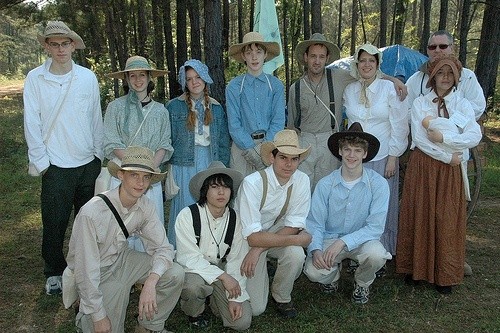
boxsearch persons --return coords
[236,130,312,316]
[61,147,185,333]
[341,42,409,275]
[285,32,408,193]
[421,115,460,133]
[23,21,103,295]
[102,55,173,254]
[410,52,483,287]
[404,30,487,275]
[174,160,253,330]
[225,33,286,184]
[163,58,232,251]
[303,122,391,305]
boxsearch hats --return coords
[295,32,341,68]
[327,121,380,163]
[107,146,167,184]
[36,21,86,49]
[105,56,172,79]
[349,43,383,109]
[228,32,281,64]
[188,161,243,202]
[261,129,311,168]
[178,59,213,92]
[426,54,463,118]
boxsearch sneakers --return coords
[46,275,62,295]
[319,281,338,293]
[352,278,370,304]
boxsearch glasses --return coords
[428,42,453,51]
[45,41,73,48]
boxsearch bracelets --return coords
[441,134,444,143]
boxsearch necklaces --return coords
[205,204,228,259]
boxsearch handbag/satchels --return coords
[28,159,40,176]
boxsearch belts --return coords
[251,134,264,139]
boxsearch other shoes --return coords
[269,290,298,318]
[462,262,473,275]
[346,257,359,272]
[133,324,175,333]
[188,316,210,328]
[417,279,430,288]
[437,285,452,293]
[377,264,387,278]
